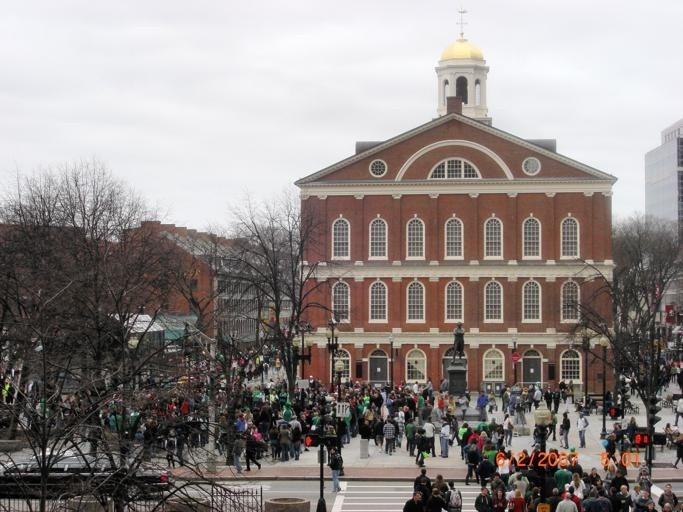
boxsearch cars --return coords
[1,447,170,500]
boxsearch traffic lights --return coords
[632,432,647,445]
[649,395,662,426]
[304,434,319,447]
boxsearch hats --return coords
[403,406,409,412]
[470,438,478,443]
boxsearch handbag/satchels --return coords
[339,466,344,476]
[528,500,535,509]
[508,422,513,430]
[507,501,514,511]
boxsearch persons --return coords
[0,334,681,512]
[451,321,465,364]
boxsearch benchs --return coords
[577,393,640,416]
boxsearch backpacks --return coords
[447,489,462,509]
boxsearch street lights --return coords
[292,322,315,414]
[389,333,395,393]
[599,330,609,440]
[511,333,519,388]
[335,356,345,462]
[325,318,342,394]
[582,326,591,417]
